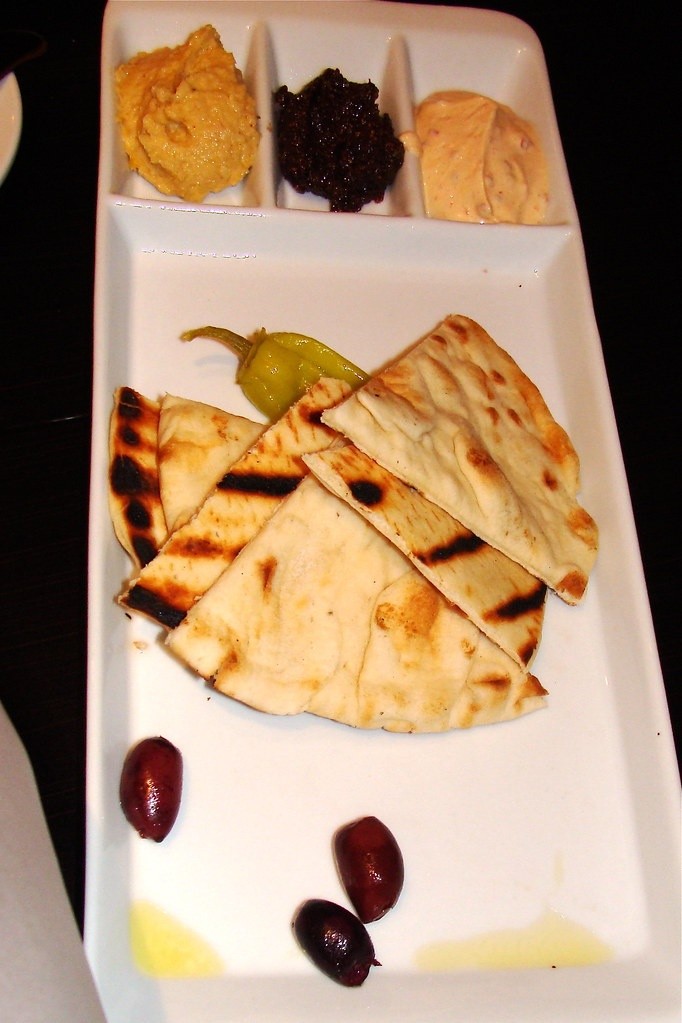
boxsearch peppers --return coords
[180,324,371,427]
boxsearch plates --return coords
[80,0,682,1023]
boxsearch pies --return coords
[105,312,599,732]
